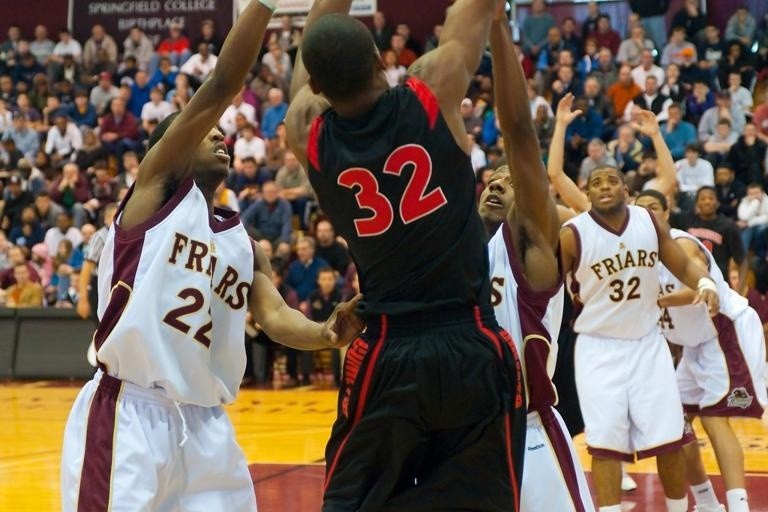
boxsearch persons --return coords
[558,163,719,512]
[633,185,768,512]
[278,0,529,512]
[475,0,595,512]
[548,280,588,442]
[539,86,683,496]
[56,0,367,512]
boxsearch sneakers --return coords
[241,375,336,386]
[598,471,637,511]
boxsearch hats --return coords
[680,47,694,55]
[31,243,48,258]
[461,98,471,106]
[98,70,113,78]
[168,24,182,30]
[7,175,20,185]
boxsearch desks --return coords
[2,305,100,381]
